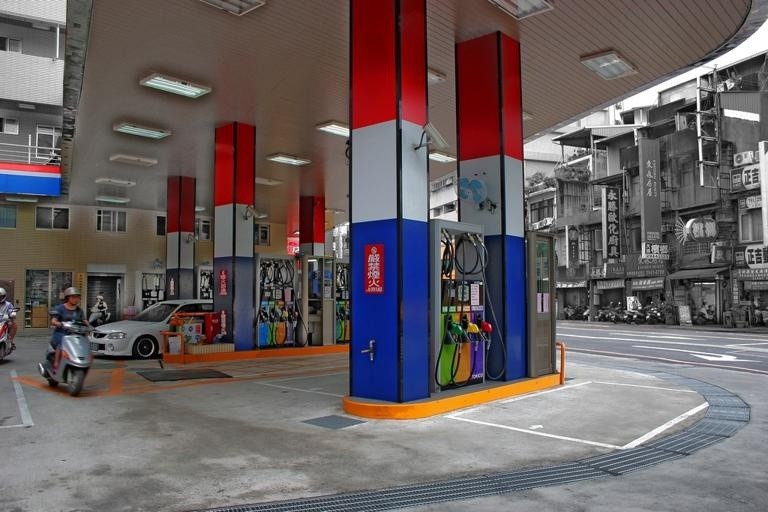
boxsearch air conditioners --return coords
[732,151,754,167]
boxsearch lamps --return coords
[94,72,213,205]
[255,119,348,186]
[491,1,555,21]
[428,150,456,164]
[425,68,448,89]
[521,112,536,122]
[580,50,640,81]
[199,0,265,18]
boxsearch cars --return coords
[89,297,215,358]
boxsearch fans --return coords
[452,176,497,214]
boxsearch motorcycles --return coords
[86,308,112,326]
[693,301,717,326]
[38,318,96,395]
[0,297,20,362]
[564,297,667,325]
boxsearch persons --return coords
[1,288,16,348]
[49,285,87,366]
[92,295,108,322]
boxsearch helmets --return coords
[63,287,81,298]
[0,287,7,302]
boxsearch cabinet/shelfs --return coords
[25,302,31,328]
[31,306,48,328]
